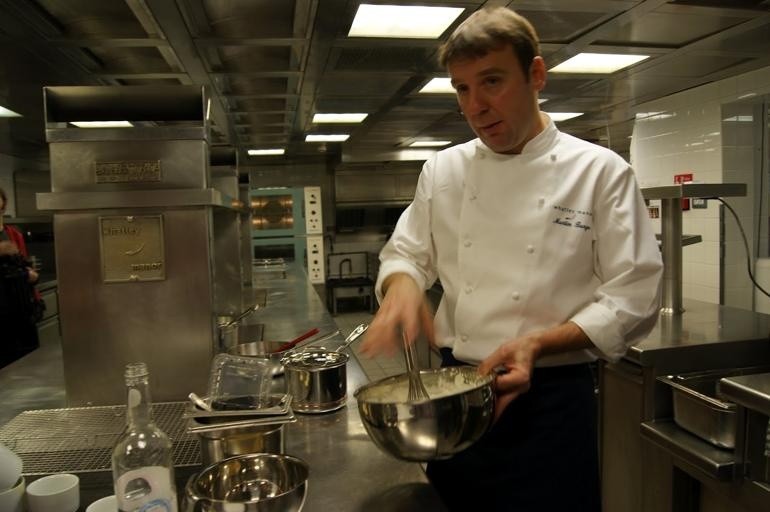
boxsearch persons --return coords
[356,6,667,511]
[0,186,42,302]
[0,215,42,369]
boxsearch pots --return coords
[228,331,338,376]
[218,304,265,350]
[282,323,369,413]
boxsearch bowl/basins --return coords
[353,366,496,462]
[181,452,310,512]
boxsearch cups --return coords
[26,473,80,512]
[0,477,27,512]
[0,444,22,490]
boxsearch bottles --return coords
[112,363,178,512]
